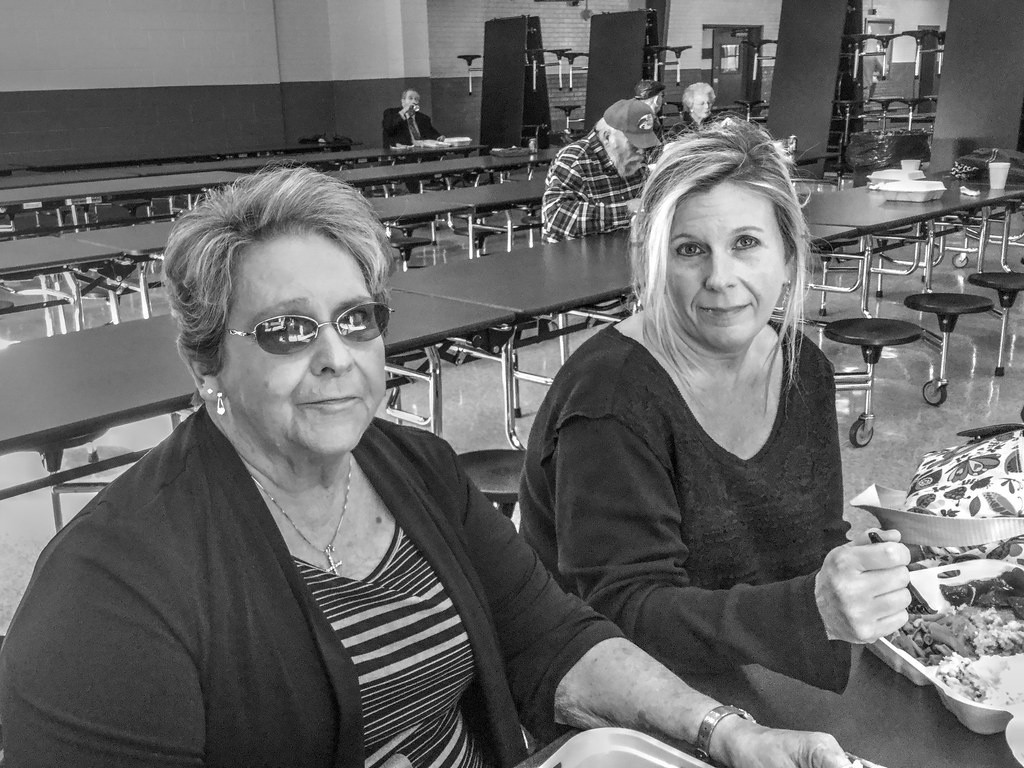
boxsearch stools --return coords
[1,28,1024,520]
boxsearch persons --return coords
[541,98,663,249]
[633,79,666,163]
[382,88,446,194]
[518,117,914,693]
[667,82,720,139]
[0,165,860,768]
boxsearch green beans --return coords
[888,608,974,664]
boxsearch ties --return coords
[407,117,420,140]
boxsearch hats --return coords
[633,80,666,99]
[606,98,661,149]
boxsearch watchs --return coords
[695,704,757,763]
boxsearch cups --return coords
[989,162,1011,190]
[899,159,922,171]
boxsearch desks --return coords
[765,0,849,179]
[0,133,362,175]
[0,146,565,241]
[927,0,1024,175]
[583,9,647,137]
[0,175,547,338]
[0,139,475,190]
[1,170,1024,520]
[478,14,528,157]
[512,640,1022,768]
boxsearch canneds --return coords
[529,138,538,153]
[413,104,420,112]
[789,135,797,152]
[631,210,645,244]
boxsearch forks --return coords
[869,531,938,621]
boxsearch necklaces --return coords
[250,451,353,576]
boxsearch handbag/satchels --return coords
[849,422,1024,566]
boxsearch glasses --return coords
[225,299,395,356]
[660,92,666,98]
